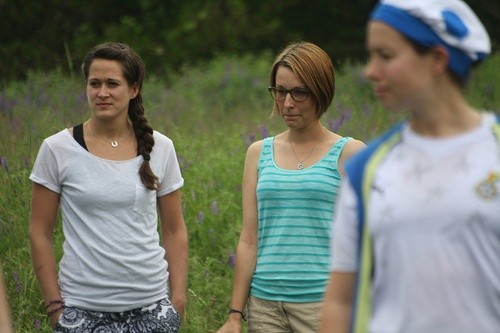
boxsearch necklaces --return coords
[287,126,326,170]
[86,118,132,147]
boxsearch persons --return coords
[0,264,12,333]
[216,41,368,333]
[29,42,189,333]
[320,0,500,333]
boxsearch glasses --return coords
[268,87,313,102]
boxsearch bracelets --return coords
[229,310,247,321]
[48,306,65,316]
[45,300,64,309]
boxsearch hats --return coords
[370,1,490,85]
